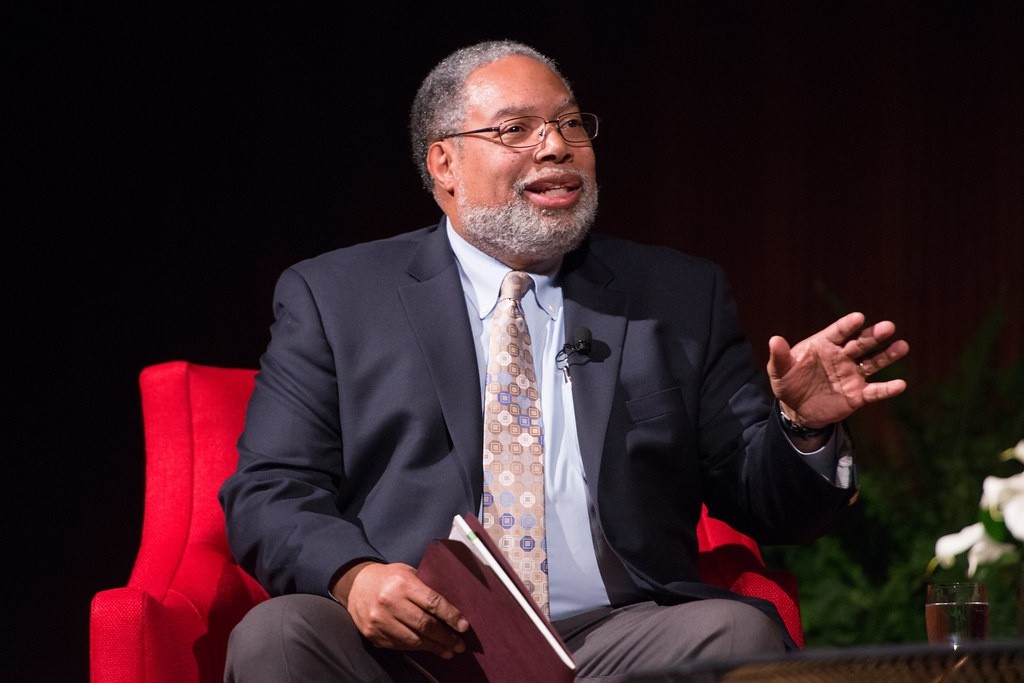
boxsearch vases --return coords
[925,582,988,646]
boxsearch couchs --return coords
[88,362,261,682]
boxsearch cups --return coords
[925,582,991,647]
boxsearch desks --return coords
[619,641,1024,683]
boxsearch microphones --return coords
[573,327,592,354]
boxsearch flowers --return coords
[930,443,1024,600]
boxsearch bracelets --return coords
[776,401,830,441]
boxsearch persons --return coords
[218,39,910,683]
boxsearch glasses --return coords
[441,112,602,148]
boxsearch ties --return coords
[483,272,552,629]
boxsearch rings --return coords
[859,359,871,376]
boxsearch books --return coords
[397,511,576,683]
